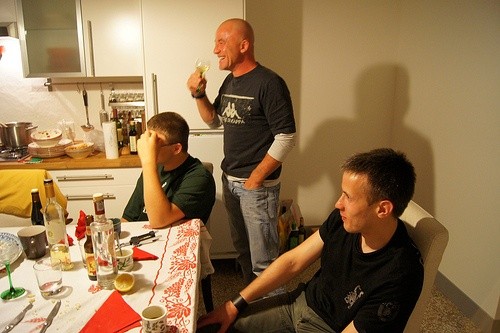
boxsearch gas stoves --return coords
[0,142,30,162]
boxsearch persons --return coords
[195,148,424,333]
[120,113,216,229]
[188,18,296,301]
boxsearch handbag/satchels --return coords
[277,199,297,253]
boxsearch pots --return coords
[0,121,38,147]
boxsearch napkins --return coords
[132,247,158,261]
[79,290,142,333]
[75,209,88,239]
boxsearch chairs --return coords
[399,200,449,333]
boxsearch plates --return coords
[26,139,72,158]
[0,231,23,273]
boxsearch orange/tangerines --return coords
[114,273,135,293]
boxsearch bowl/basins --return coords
[65,143,94,160]
[31,130,63,146]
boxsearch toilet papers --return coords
[102,120,119,160]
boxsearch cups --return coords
[141,305,167,333]
[115,249,134,270]
[18,225,46,258]
[107,218,121,239]
[79,238,88,268]
[33,257,63,296]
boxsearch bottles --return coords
[90,193,119,290]
[30,189,45,225]
[42,179,72,268]
[83,215,93,281]
[112,107,146,155]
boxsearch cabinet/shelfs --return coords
[47,0,247,275]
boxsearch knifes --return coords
[40,299,60,333]
[2,303,32,333]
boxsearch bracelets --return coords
[230,292,248,313]
[192,92,206,99]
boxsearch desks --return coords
[0,218,213,333]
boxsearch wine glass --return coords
[0,240,26,300]
[190,58,210,94]
